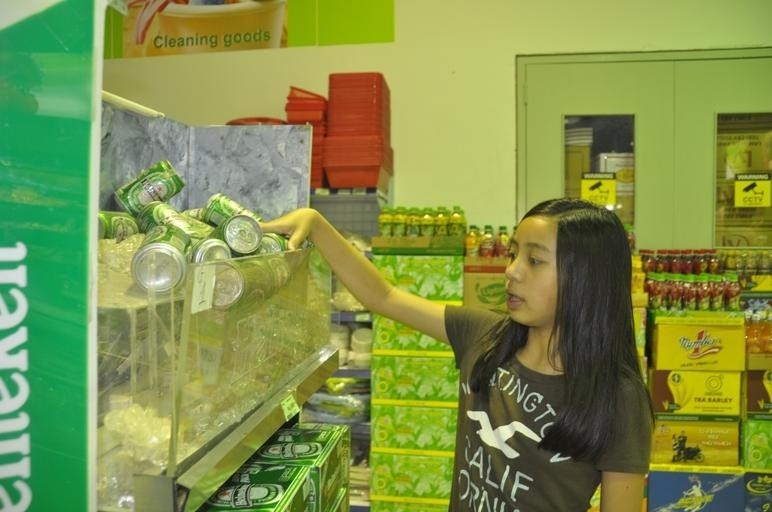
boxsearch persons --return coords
[254,194,654,512]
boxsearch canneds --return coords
[98,159,299,308]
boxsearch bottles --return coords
[639,248,771,310]
[377,206,517,258]
[742,298,772,354]
[624,224,635,256]
